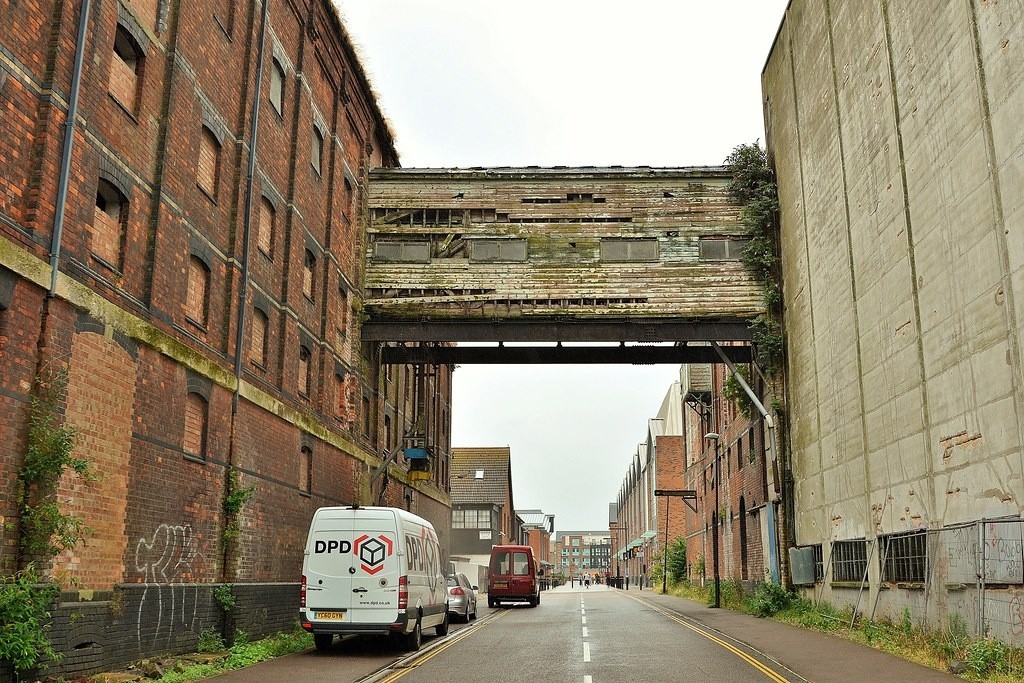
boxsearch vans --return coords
[488,544,544,608]
[300,506,455,652]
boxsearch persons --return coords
[584,572,589,589]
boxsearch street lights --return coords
[704,432,720,609]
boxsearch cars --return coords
[446,571,478,624]
